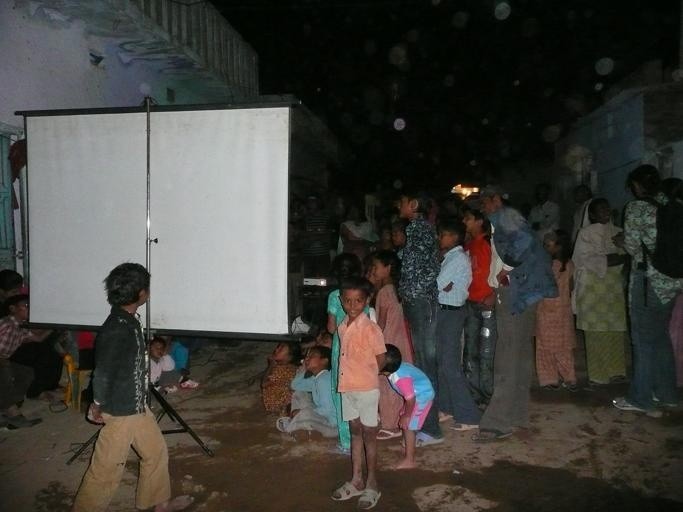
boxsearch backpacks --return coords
[636,193,683,280]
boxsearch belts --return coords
[439,304,461,312]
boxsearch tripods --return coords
[65,99,215,464]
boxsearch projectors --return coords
[303,275,333,286]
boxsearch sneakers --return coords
[179,380,200,390]
[611,396,664,420]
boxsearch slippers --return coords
[357,488,383,511]
[471,427,512,442]
[401,432,447,450]
[376,427,403,440]
[329,480,365,502]
[439,412,480,432]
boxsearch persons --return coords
[149,337,174,385]
[0,270,22,302]
[331,276,386,511]
[71,263,194,511]
[156,335,199,394]
[48,328,71,377]
[0,294,54,430]
